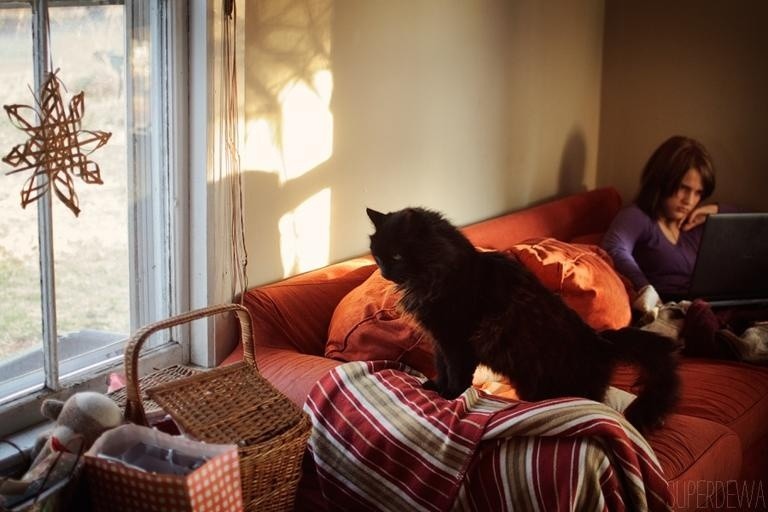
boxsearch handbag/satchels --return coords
[86,425,244,512]
[0,434,86,512]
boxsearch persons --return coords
[601,136,756,312]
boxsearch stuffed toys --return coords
[0,392,124,512]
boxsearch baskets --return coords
[102,303,314,512]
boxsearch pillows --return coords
[505,230,638,334]
[316,242,499,393]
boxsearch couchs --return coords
[217,184,767,512]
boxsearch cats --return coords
[364,204,683,434]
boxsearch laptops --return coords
[675,212,768,308]
